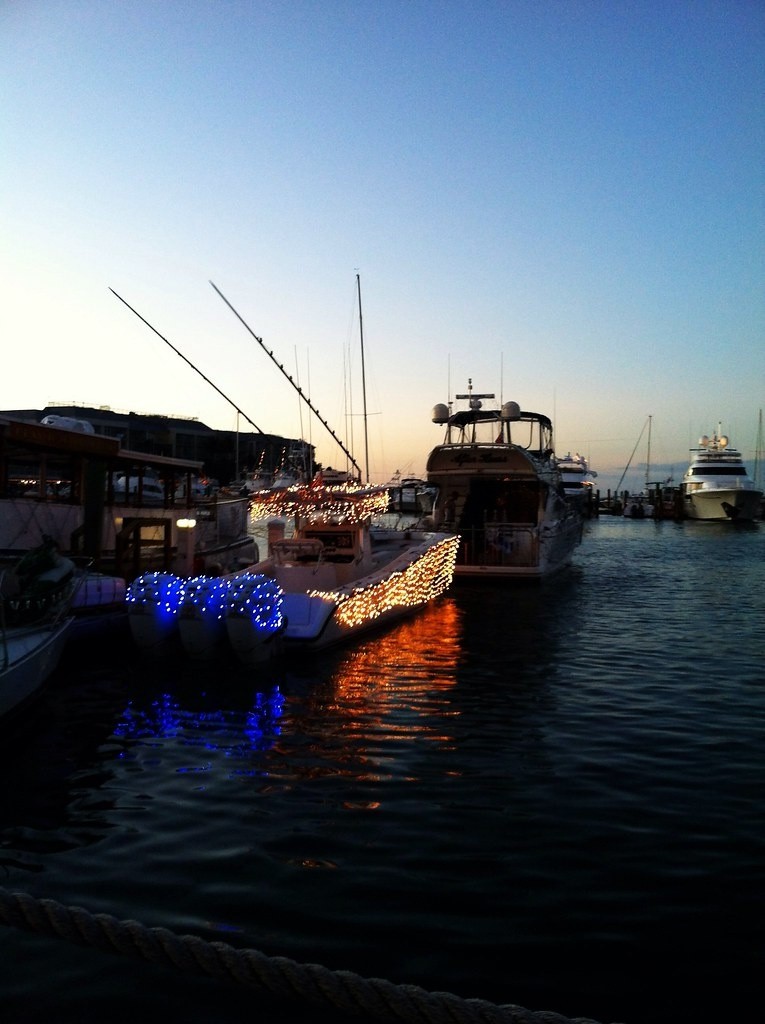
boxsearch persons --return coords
[439,491,457,537]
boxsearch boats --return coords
[0,280,765,726]
